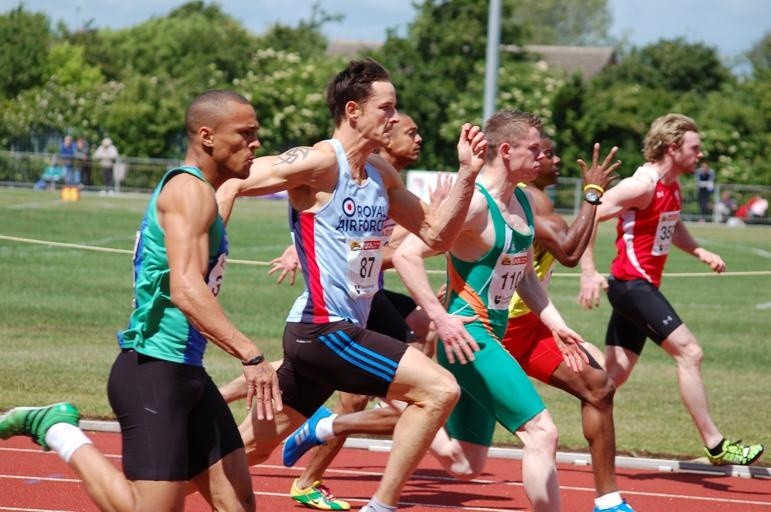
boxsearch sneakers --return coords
[282,404,333,466]
[704,439,764,466]
[0,401,81,451]
[594,497,634,512]
[290,478,350,511]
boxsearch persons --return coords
[695,161,768,223]
[0,87,282,511]
[578,113,765,464]
[33,132,118,194]
[215,57,634,511]
